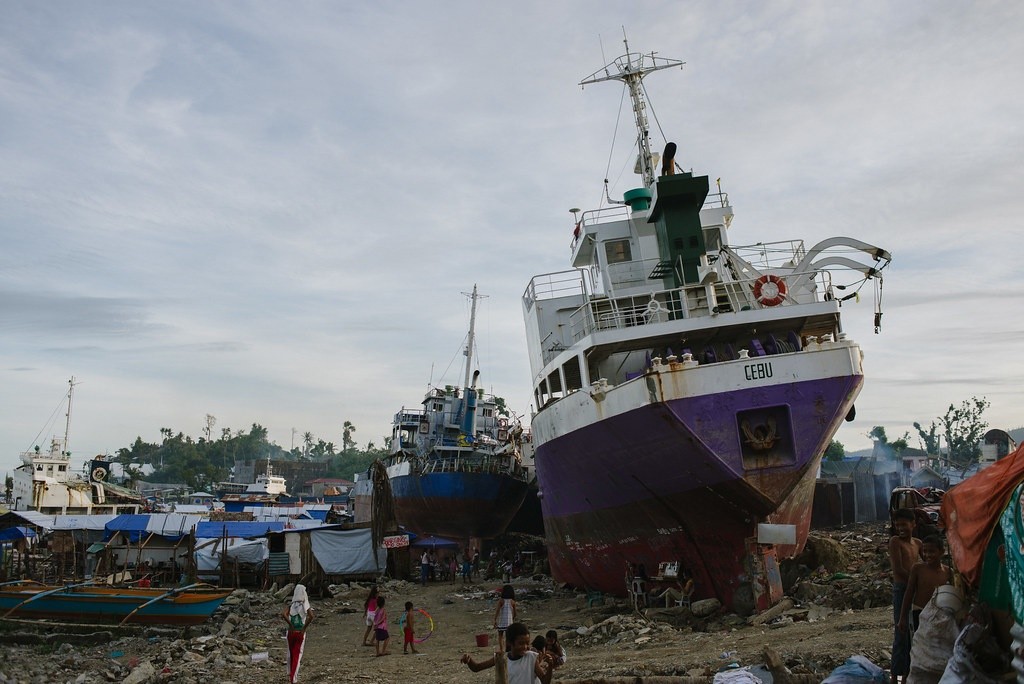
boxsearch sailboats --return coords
[245,453,287,496]
[11,375,140,517]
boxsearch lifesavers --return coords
[752,275,786,306]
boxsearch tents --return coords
[413,538,460,563]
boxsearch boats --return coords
[0,577,236,627]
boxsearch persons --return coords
[530,630,566,671]
[421,545,516,586]
[404,601,419,654]
[169,558,178,581]
[494,585,517,654]
[896,535,954,637]
[373,597,390,656]
[121,507,132,514]
[96,509,101,514]
[255,559,269,589]
[283,585,314,684]
[461,622,553,684]
[145,561,152,571]
[889,508,926,684]
[138,498,158,514]
[362,585,378,646]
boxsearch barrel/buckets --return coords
[476,634,488,647]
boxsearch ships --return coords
[522,23,895,616]
[370,282,541,584]
